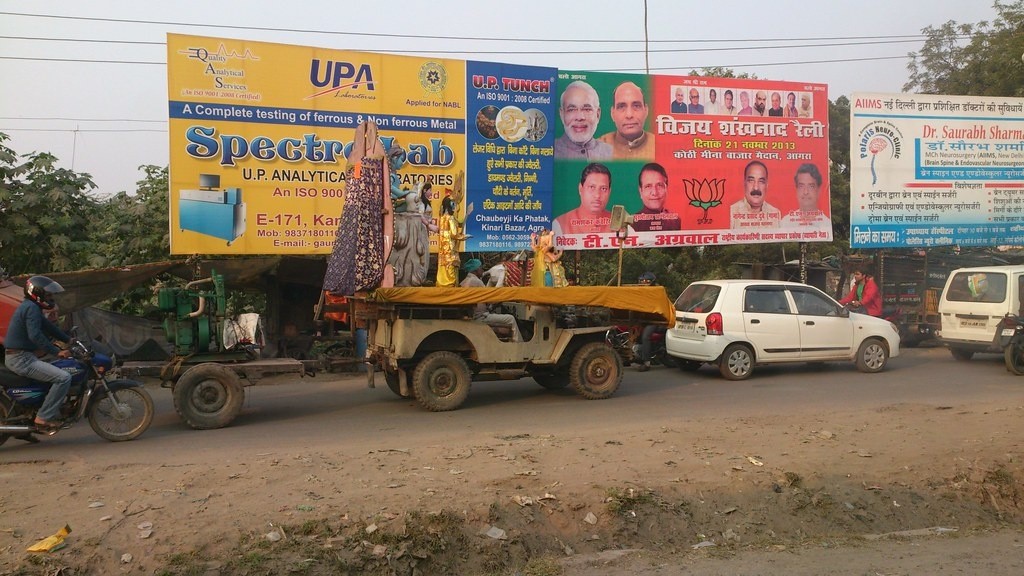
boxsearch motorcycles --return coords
[838,301,901,330]
[1,326,153,447]
[605,320,680,368]
[1000,312,1024,375]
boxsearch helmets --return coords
[638,271,656,286]
[24,276,57,310]
[42,304,59,325]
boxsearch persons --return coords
[730,159,781,230]
[550,162,636,233]
[3,273,71,428]
[596,80,655,162]
[460,257,524,342]
[780,162,831,230]
[622,163,681,232]
[671,86,812,118]
[837,266,885,318]
[634,269,664,371]
[438,195,473,286]
[414,177,438,285]
[553,80,611,161]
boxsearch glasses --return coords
[691,96,699,99]
[564,108,593,115]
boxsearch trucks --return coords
[877,246,1011,349]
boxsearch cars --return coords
[666,277,901,381]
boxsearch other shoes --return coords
[639,362,650,371]
[34,417,64,427]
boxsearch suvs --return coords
[934,264,1024,362]
[363,255,632,410]
